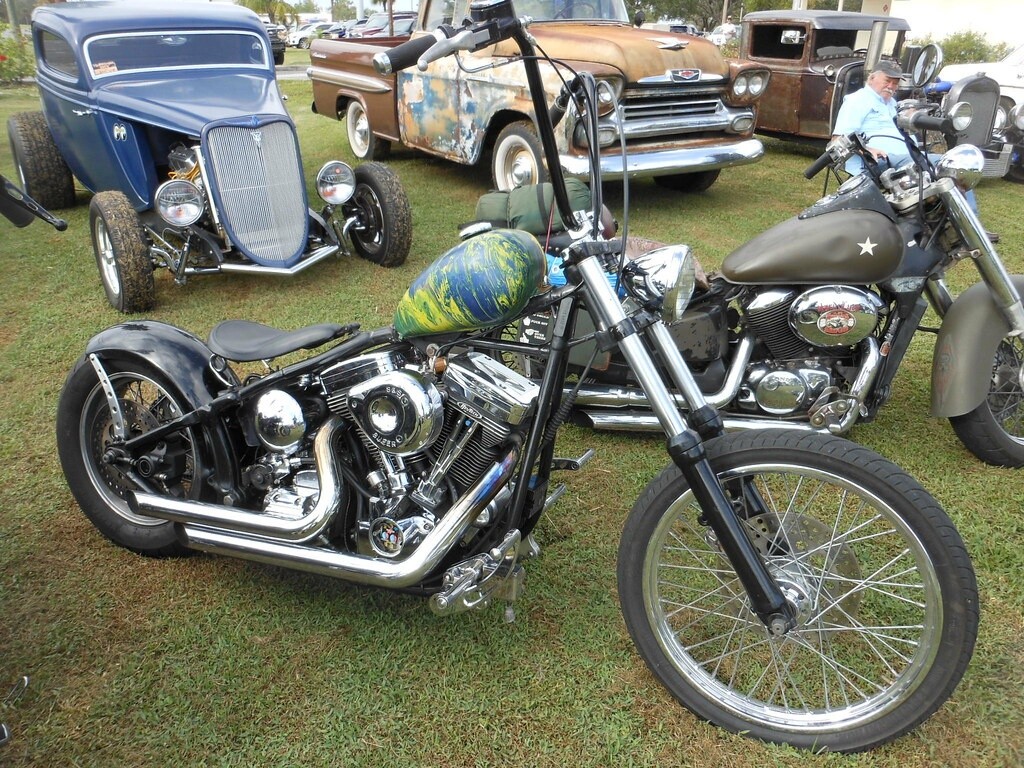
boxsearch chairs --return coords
[822,61,867,201]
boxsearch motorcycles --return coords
[56,0,981,754]
[474,99,1023,469]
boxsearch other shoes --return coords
[984,230,999,242]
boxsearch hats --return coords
[727,15,732,20]
[872,60,906,82]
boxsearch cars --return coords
[711,26,743,46]
[739,10,1024,178]
[252,24,287,65]
[6,1,412,314]
[288,11,418,49]
[936,43,1024,136]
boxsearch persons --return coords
[721,15,737,45]
[831,60,1000,242]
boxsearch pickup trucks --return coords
[306,0,772,193]
